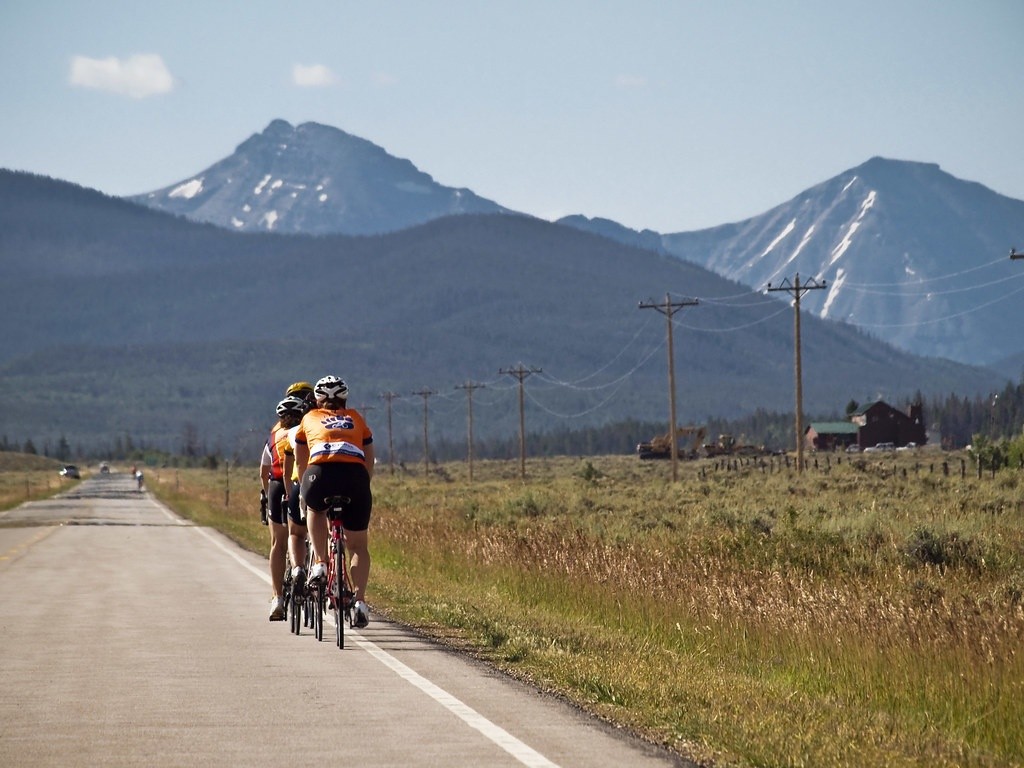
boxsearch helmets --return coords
[276,395,309,418]
[286,381,313,398]
[313,375,349,400]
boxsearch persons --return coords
[132,463,143,488]
[260,375,374,628]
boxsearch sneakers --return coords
[293,567,306,595]
[306,562,328,588]
[269,595,285,619]
[353,601,369,629]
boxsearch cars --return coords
[60,465,81,480]
[896,442,918,451]
[100,461,110,473]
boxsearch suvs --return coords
[863,443,896,455]
[846,444,863,453]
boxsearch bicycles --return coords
[138,477,144,489]
[260,489,358,650]
